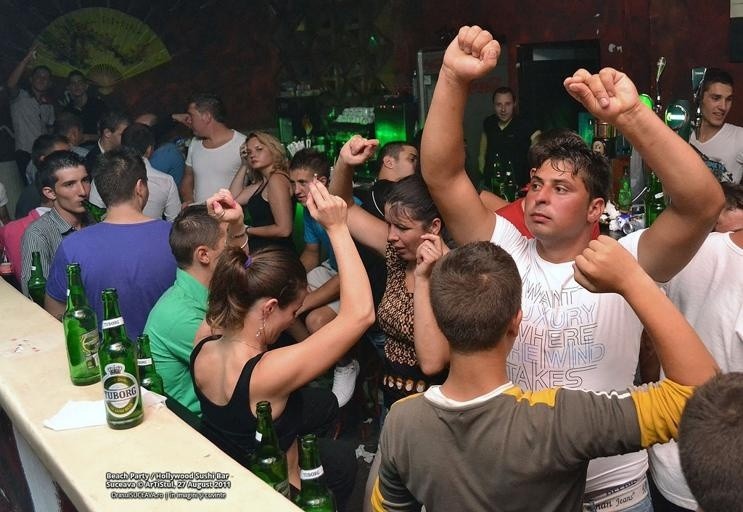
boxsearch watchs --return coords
[226,225,245,239]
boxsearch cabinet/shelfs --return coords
[0,278,308,511]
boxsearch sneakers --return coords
[331,359,360,407]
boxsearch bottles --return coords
[617,166,631,214]
[249,401,290,501]
[502,162,519,202]
[336,107,374,124]
[0,246,15,287]
[342,142,345,149]
[27,252,46,309]
[62,262,101,386]
[98,288,144,430]
[363,160,371,179]
[650,179,665,228]
[295,433,337,512]
[490,153,503,199]
[175,137,184,152]
[329,141,337,166]
[318,137,325,153]
[134,333,164,397]
[81,199,106,223]
[644,177,656,228]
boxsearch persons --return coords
[420,25,726,512]
[0,172,54,285]
[44,145,177,338]
[687,66,742,184]
[189,178,376,457]
[268,147,375,407]
[21,151,97,297]
[83,112,130,172]
[87,122,182,224]
[0,180,12,226]
[16,133,72,217]
[9,41,56,187]
[328,136,452,409]
[56,71,111,129]
[478,87,541,201]
[674,366,742,512]
[639,183,743,512]
[132,114,184,184]
[351,140,416,276]
[233,131,297,264]
[22,113,91,185]
[138,205,228,433]
[371,235,721,512]
[157,113,192,133]
[183,94,249,207]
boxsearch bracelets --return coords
[245,226,250,233]
[224,239,248,249]
